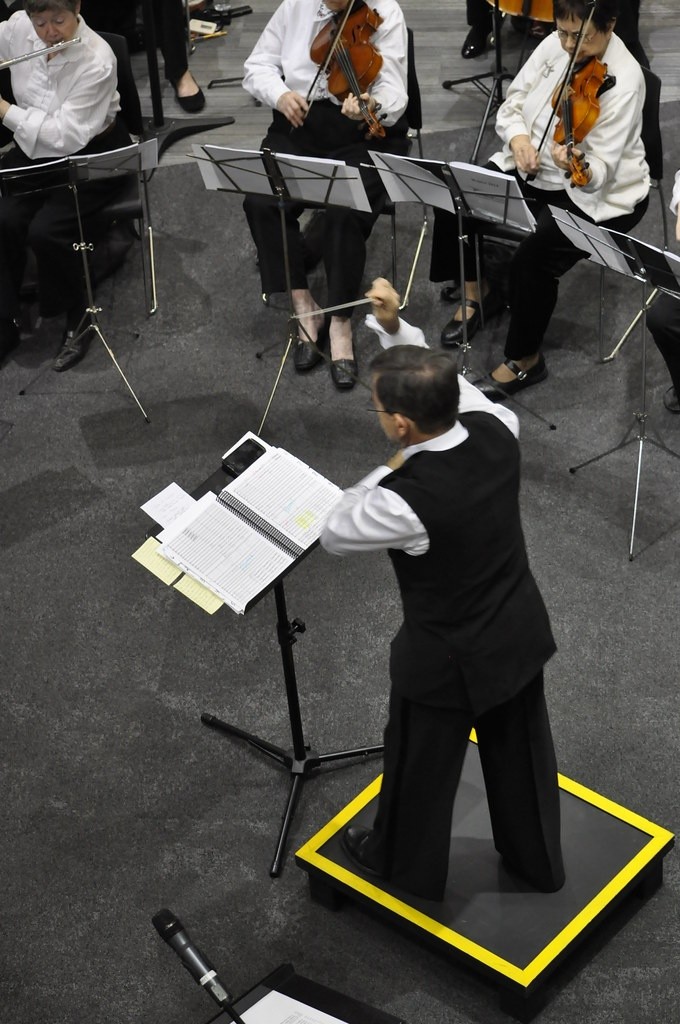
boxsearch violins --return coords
[308,0,388,142]
[552,55,608,190]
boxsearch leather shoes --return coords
[0,318,22,361]
[53,320,97,373]
[461,25,492,58]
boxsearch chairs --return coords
[261,28,427,310]
[85,31,157,314]
[470,67,668,363]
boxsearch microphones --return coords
[151,909,233,1007]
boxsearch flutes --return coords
[0,36,81,71]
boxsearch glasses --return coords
[557,30,597,44]
[364,398,401,416]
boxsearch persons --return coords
[319,277,566,900]
[441,0,680,403]
[242,0,409,388]
[0,0,205,371]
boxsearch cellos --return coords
[484,0,596,75]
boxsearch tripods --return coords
[144,436,386,877]
[443,0,517,167]
[359,153,557,431]
[187,147,373,436]
[551,209,680,561]
[0,152,150,426]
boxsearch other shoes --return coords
[340,824,388,879]
[440,283,507,344]
[293,315,357,388]
[471,352,548,403]
[164,65,205,113]
